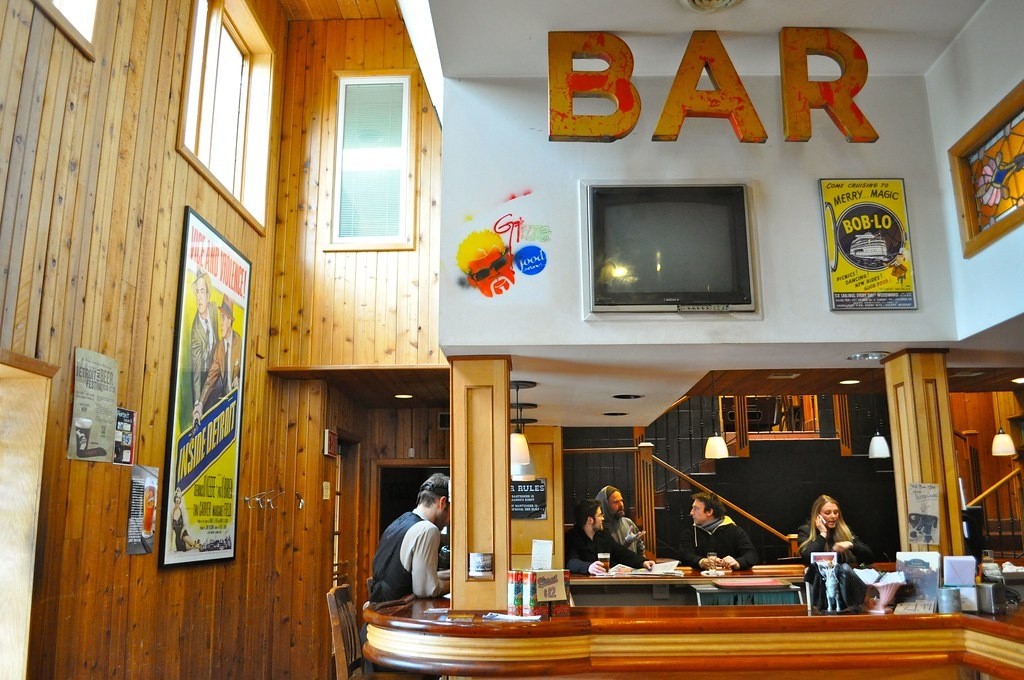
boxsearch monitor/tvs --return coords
[591,185,755,312]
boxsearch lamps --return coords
[705,395,729,459]
[992,367,1016,456]
[509,380,538,482]
[868,395,890,459]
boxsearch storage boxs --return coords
[507,568,571,619]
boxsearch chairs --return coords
[326,583,443,680]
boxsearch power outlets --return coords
[653,584,669,599]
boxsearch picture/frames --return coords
[510,478,547,520]
[438,412,450,430]
[157,204,253,570]
[818,176,919,312]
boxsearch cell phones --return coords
[818,513,826,525]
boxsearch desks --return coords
[690,584,804,606]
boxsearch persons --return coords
[190,268,218,426]
[564,498,655,576]
[359,473,451,673]
[797,494,871,566]
[170,486,203,552]
[678,492,758,571]
[593,486,647,560]
[192,291,243,419]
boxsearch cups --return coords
[76,418,92,458]
[981,550,994,563]
[938,587,960,614]
[598,553,610,574]
[141,476,157,537]
[708,552,717,575]
